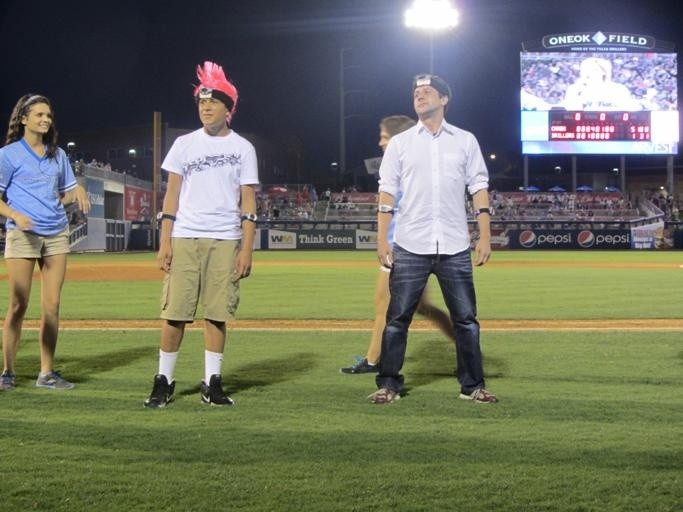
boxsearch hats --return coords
[194,80,238,112]
[413,74,451,102]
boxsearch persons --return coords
[142,61,260,408]
[89,158,112,172]
[339,115,455,375]
[0,95,91,390]
[465,192,633,223]
[521,50,677,111]
[365,74,500,405]
[257,185,380,227]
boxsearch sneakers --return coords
[371,388,401,404]
[459,388,498,404]
[200,374,234,406]
[0,369,15,390]
[341,358,381,375]
[36,370,75,390]
[143,374,176,408]
